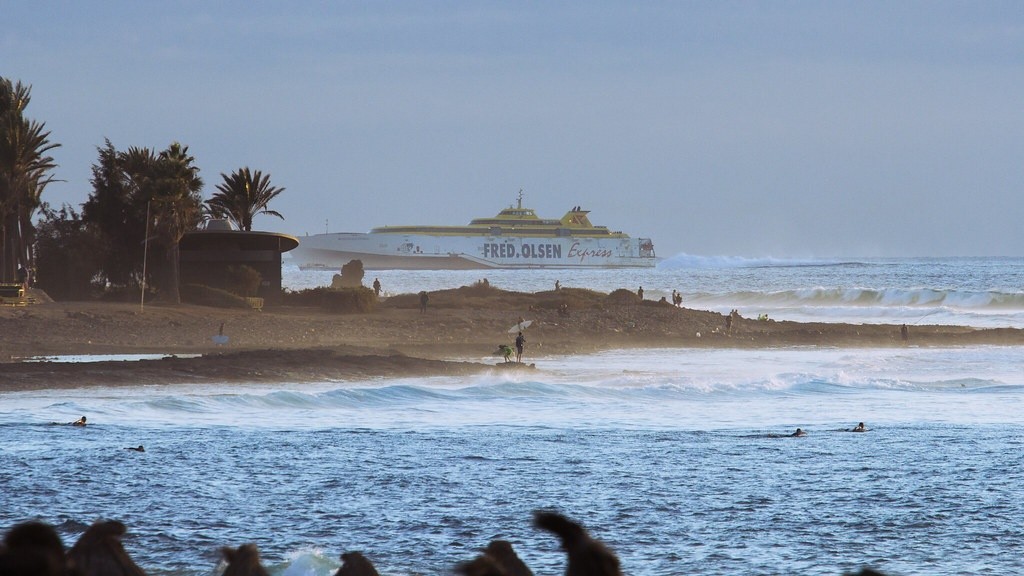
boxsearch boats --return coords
[289,189,656,269]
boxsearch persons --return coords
[787,428,807,438]
[839,422,873,432]
[2,506,627,576]
[420,291,429,313]
[219,322,224,336]
[673,289,677,305]
[517,304,571,333]
[499,345,512,362]
[901,324,908,344]
[124,446,144,452]
[555,280,561,291]
[516,331,525,361]
[77,416,86,426]
[726,309,769,338]
[638,286,644,300]
[676,292,683,308]
[373,278,381,296]
